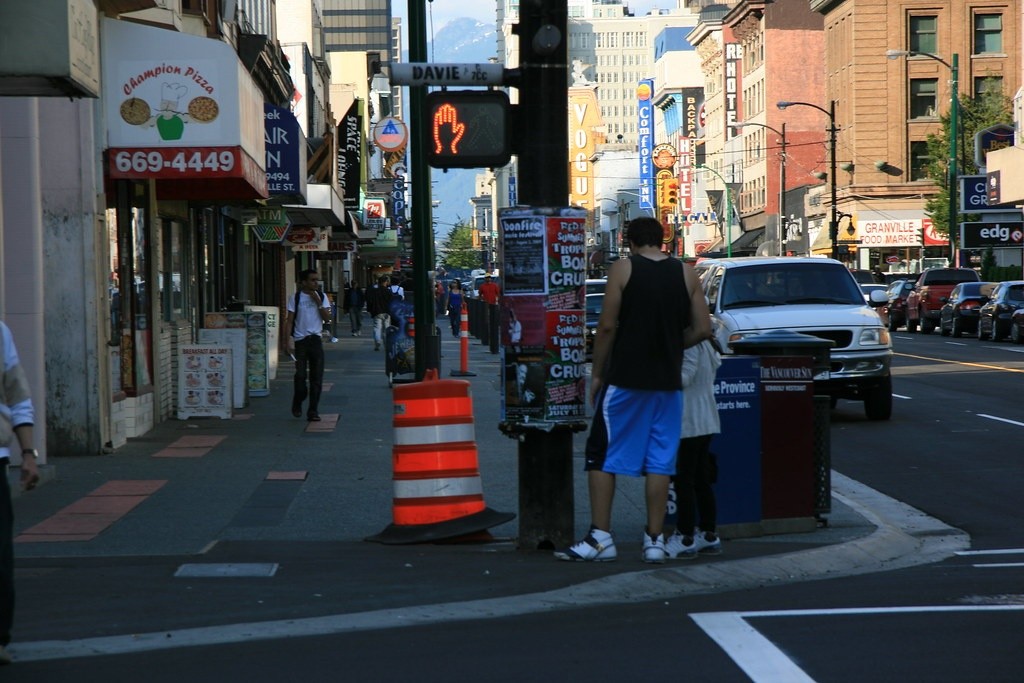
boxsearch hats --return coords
[485,273,491,277]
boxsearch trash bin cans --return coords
[653,355,761,527]
[726,327,837,530]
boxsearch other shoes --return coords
[352,330,361,337]
[307,412,321,421]
[292,401,302,417]
[374,342,381,352]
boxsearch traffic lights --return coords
[422,90,512,168]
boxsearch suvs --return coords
[883,277,916,332]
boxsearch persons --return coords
[479,272,499,304]
[874,265,884,275]
[0,323,39,663]
[284,269,331,421]
[343,280,364,336]
[553,218,712,563]
[367,274,465,352]
[665,294,722,560]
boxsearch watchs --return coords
[21,448,38,458]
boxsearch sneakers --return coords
[665,529,722,560]
[553,524,618,563]
[640,530,665,564]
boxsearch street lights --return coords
[885,49,958,269]
[610,191,656,219]
[776,102,855,260]
[728,122,786,257]
[596,198,624,260]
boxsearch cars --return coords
[978,281,1024,342]
[940,282,1000,338]
[905,268,981,334]
[463,274,608,364]
[858,282,889,311]
[1010,308,1024,345]
[694,254,893,422]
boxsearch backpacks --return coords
[389,285,402,301]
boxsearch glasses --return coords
[310,278,318,282]
[452,282,456,284]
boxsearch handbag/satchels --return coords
[366,288,389,316]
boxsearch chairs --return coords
[724,283,743,305]
